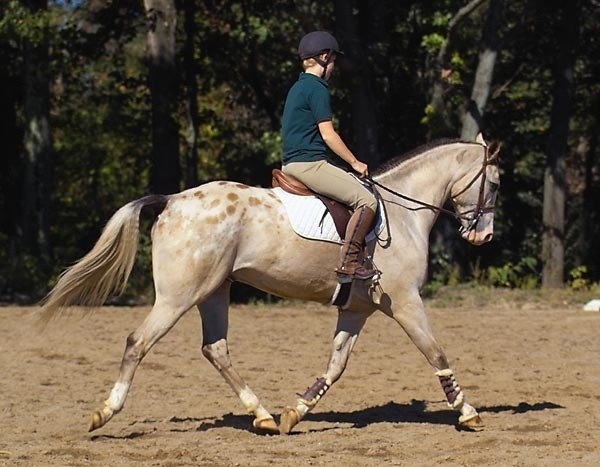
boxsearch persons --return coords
[280,31,383,281]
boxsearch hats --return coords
[298,31,344,60]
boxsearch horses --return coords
[33,131,502,434]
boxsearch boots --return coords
[336,205,382,284]
